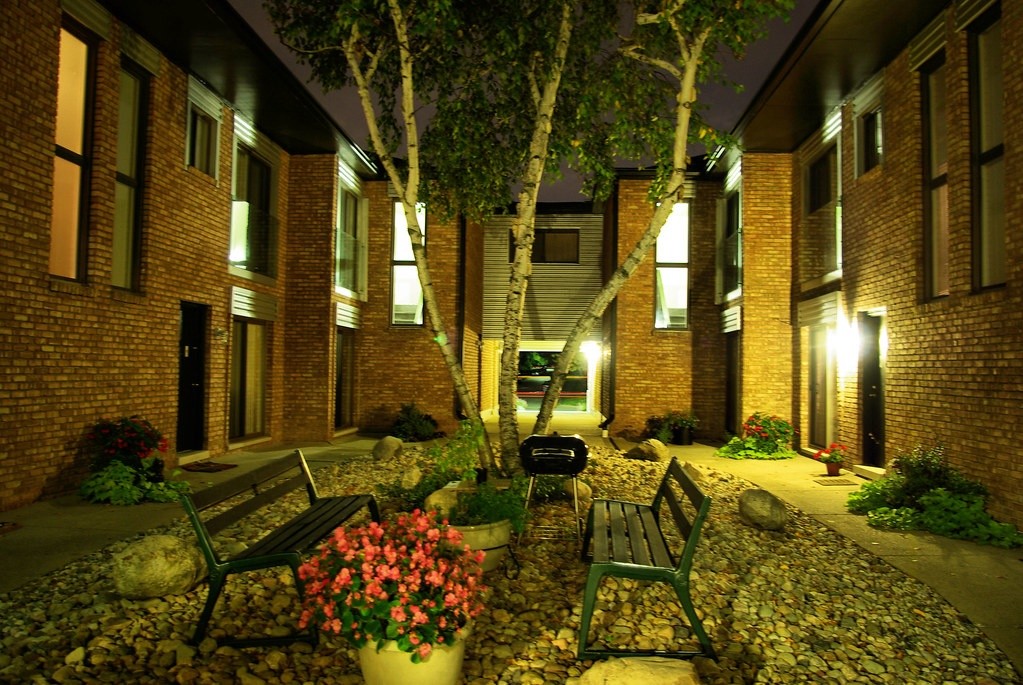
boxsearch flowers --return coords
[746,412,799,447]
[298,507,493,664]
[76,415,170,467]
[814,443,847,463]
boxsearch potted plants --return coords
[431,483,528,572]
[658,410,699,445]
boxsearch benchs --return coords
[181,449,380,651]
[577,457,720,665]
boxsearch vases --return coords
[827,462,842,475]
[123,457,144,484]
[358,624,472,685]
[757,432,779,445]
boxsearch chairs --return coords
[515,437,588,550]
